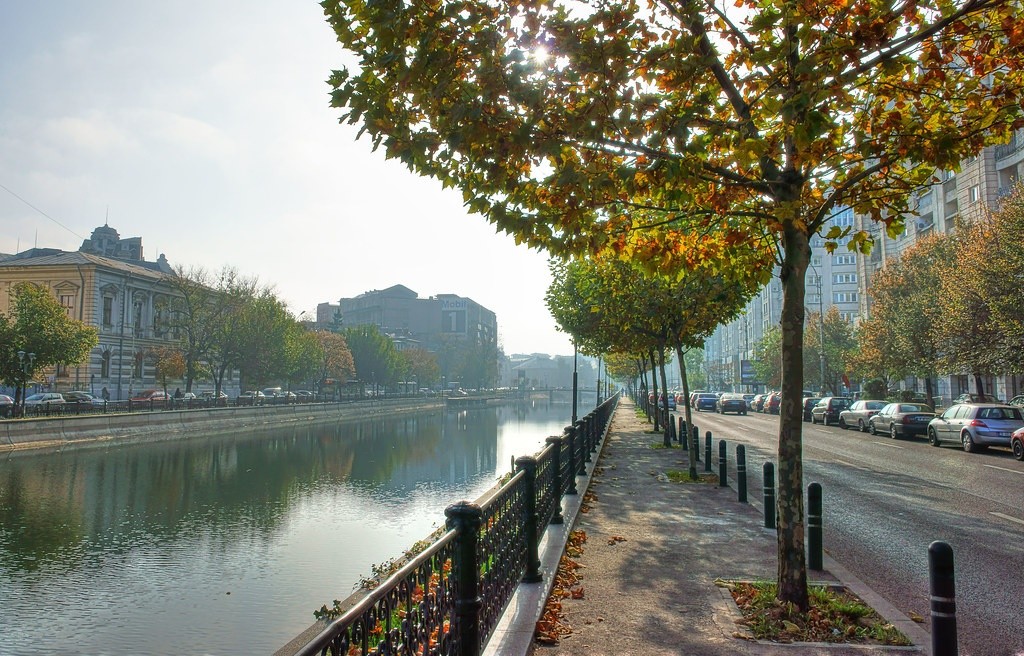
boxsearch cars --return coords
[695,393,719,411]
[130,389,228,401]
[239,388,312,404]
[1009,426,1024,460]
[1,389,103,410]
[869,401,940,439]
[715,392,747,415]
[837,400,892,432]
[810,397,857,426]
[763,393,781,412]
[418,387,468,397]
[927,396,1024,452]
[750,394,769,411]
[648,389,755,412]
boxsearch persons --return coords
[102,387,108,398]
[175,388,181,397]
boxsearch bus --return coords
[317,378,361,401]
[384,379,418,400]
